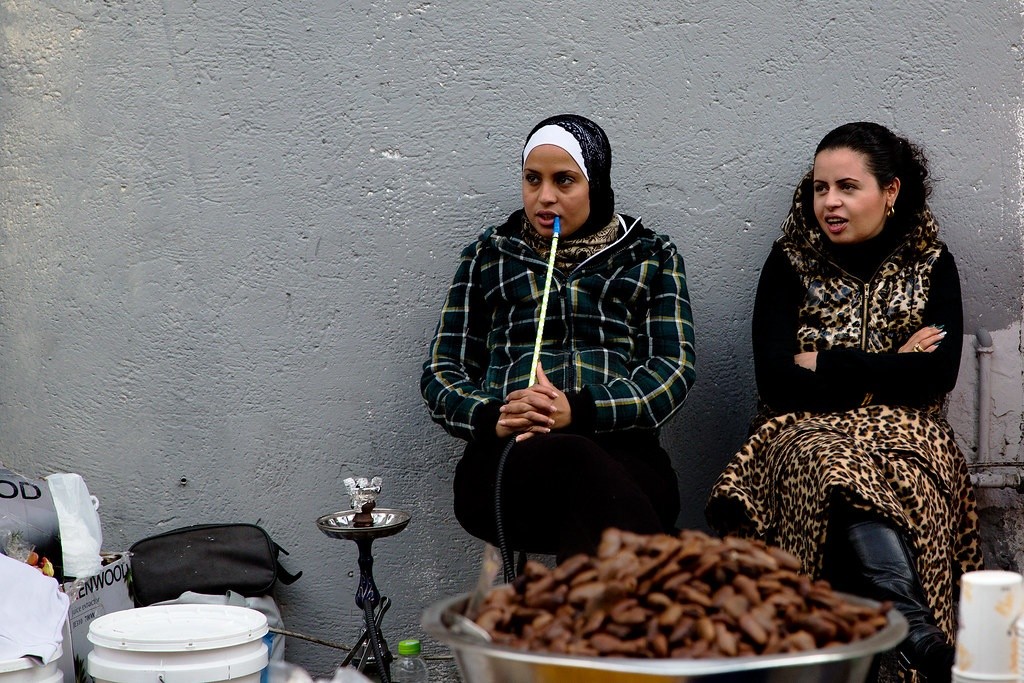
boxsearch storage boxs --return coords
[58,549,130,683]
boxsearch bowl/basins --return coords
[423,589,908,683]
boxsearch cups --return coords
[951,570,1024,683]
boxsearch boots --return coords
[832,518,955,683]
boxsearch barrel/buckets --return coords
[87,604,269,683]
[0,642,64,683]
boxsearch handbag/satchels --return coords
[127,518,302,607]
[146,589,286,683]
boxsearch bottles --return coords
[389,640,429,683]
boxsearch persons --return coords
[752,121,963,683]
[420,114,696,566]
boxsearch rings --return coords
[914,343,925,353]
